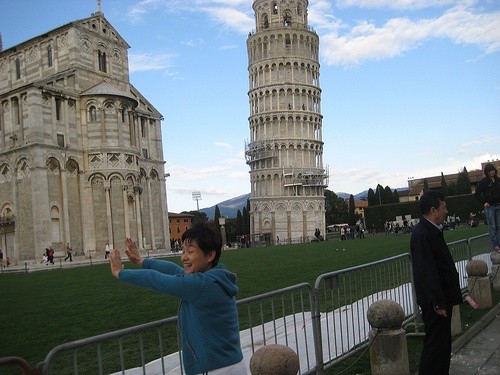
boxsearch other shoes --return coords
[494,246,500,252]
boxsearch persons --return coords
[315,228,320,239]
[385,218,408,235]
[0,250,3,269]
[170,238,181,252]
[65,243,72,262]
[341,222,365,240]
[46,248,54,265]
[410,191,464,375]
[106,223,247,375]
[240,235,249,248]
[476,164,500,253]
[450,213,459,230]
[105,243,109,259]
